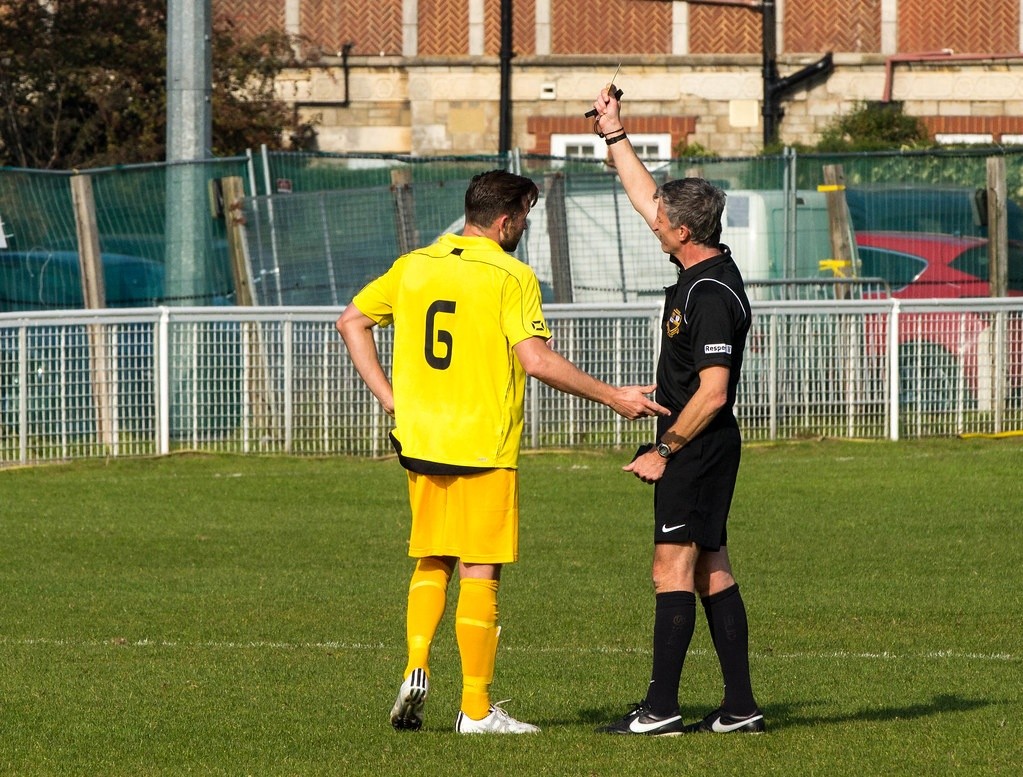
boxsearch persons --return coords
[593,82,768,737]
[334,169,672,732]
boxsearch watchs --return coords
[656,439,674,458]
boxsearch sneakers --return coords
[391,668,429,732]
[607,699,686,739]
[693,705,767,735]
[456,698,541,737]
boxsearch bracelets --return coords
[605,132,627,145]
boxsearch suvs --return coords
[845,183,1023,415]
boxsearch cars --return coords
[1,249,248,439]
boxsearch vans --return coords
[419,186,864,306]
